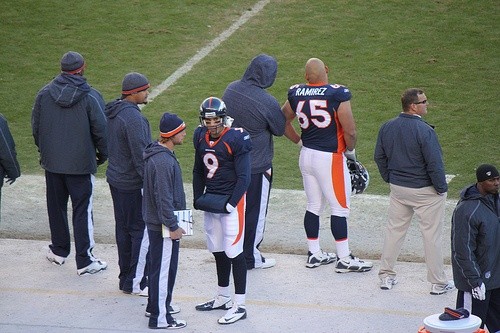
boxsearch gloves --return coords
[472,282,486,300]
[345,148,356,162]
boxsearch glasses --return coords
[414,100,427,104]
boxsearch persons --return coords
[281,58,373,274]
[222,54,287,270]
[104,72,151,296]
[451,164,500,333]
[142,112,187,328]
[192,96,251,324]
[32,51,107,275]
[0,115,21,196]
[374,88,454,294]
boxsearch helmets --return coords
[200,96,227,118]
[346,159,369,195]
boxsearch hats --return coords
[159,112,186,138]
[476,164,500,182]
[61,51,86,74]
[121,72,150,95]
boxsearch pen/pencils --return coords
[183,219,193,225]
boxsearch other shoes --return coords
[195,298,233,311]
[77,260,108,277]
[334,252,374,273]
[247,258,276,270]
[217,305,247,325]
[161,319,187,330]
[46,249,66,266]
[430,280,455,295]
[135,286,149,297]
[305,249,337,268]
[145,306,181,317]
[380,276,398,290]
